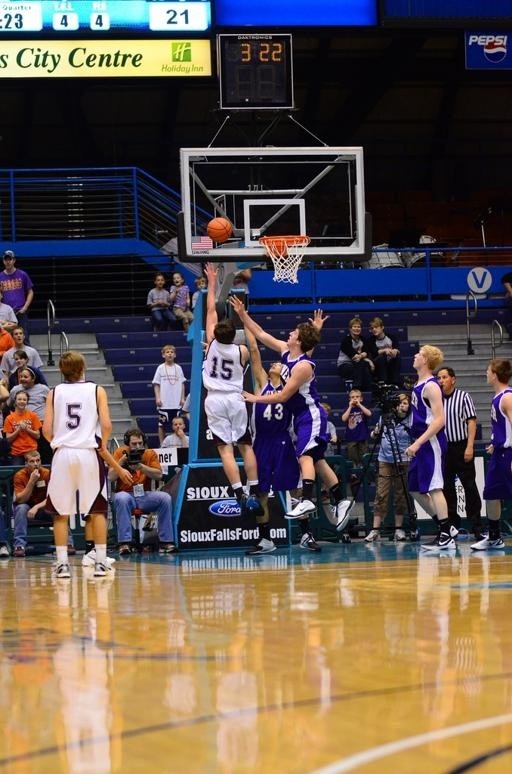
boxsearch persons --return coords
[201,261,268,522]
[225,292,358,534]
[151,344,188,445]
[434,365,487,542]
[11,448,76,557]
[243,303,330,556]
[335,314,375,387]
[232,268,254,312]
[365,315,403,388]
[468,358,512,552]
[0,248,55,467]
[40,349,117,578]
[402,343,461,552]
[319,401,338,457]
[161,415,190,449]
[79,380,136,568]
[106,428,178,555]
[1,506,12,557]
[146,271,177,338]
[190,275,208,308]
[500,270,511,340]
[169,270,195,330]
[340,387,374,467]
[181,392,191,421]
[362,392,413,543]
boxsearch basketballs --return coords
[207,218,232,243]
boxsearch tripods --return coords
[334,410,421,542]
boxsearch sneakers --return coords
[51,561,74,579]
[244,533,279,556]
[419,520,506,551]
[283,498,317,521]
[0,545,10,559]
[157,541,178,553]
[80,547,118,577]
[363,526,382,543]
[393,527,407,541]
[119,544,132,555]
[332,497,358,531]
[67,544,77,555]
[236,493,266,522]
[13,546,26,557]
[299,531,321,552]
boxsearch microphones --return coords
[402,410,410,413]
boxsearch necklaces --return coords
[164,363,177,385]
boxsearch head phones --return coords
[124,430,149,446]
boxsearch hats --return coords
[2,250,15,258]
